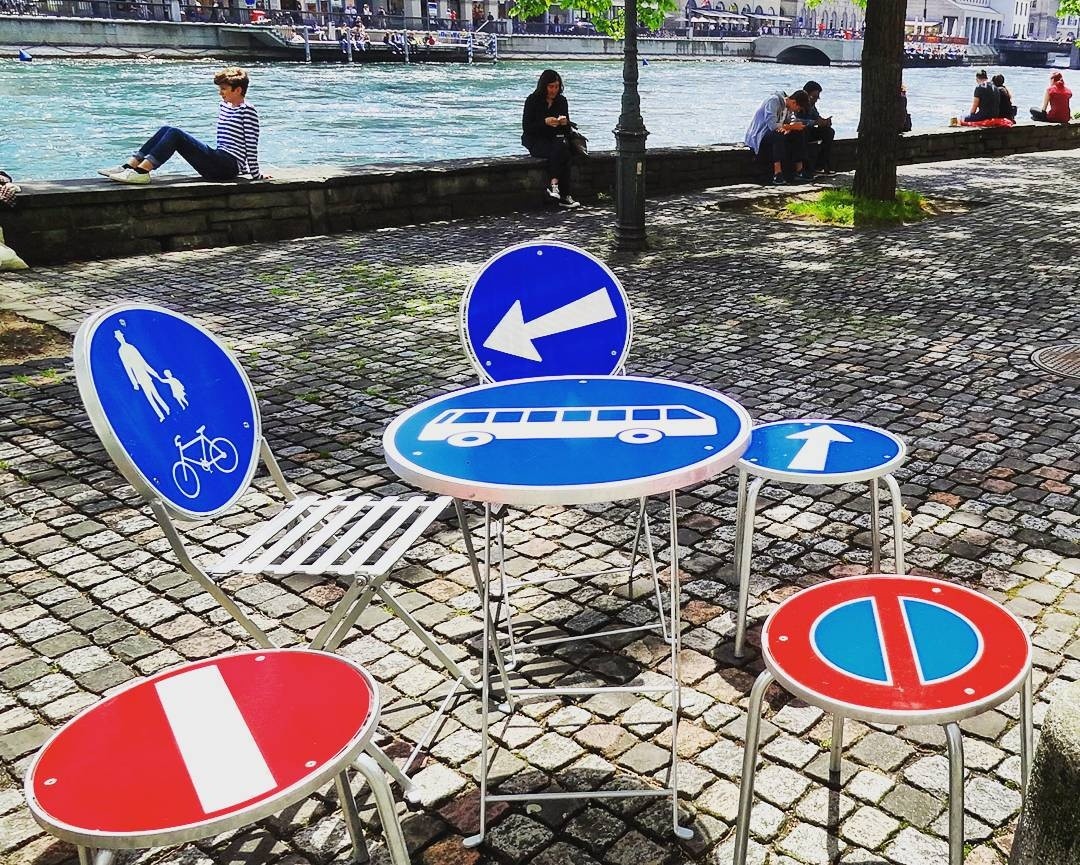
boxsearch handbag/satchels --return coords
[566,131,589,155]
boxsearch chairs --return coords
[460,243,679,672]
[72,301,477,804]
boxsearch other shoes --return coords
[812,168,837,176]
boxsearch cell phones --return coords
[827,116,833,119]
[557,116,567,121]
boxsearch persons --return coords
[964,70,1014,121]
[744,81,835,185]
[900,85,907,132]
[521,70,580,207]
[706,23,862,40]
[0,0,590,54]
[1031,71,1072,122]
[97,67,273,184]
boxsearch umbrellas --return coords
[664,14,748,31]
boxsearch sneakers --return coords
[546,184,561,199]
[792,168,816,182]
[97,165,126,177]
[772,172,786,185]
[109,168,152,184]
[558,195,580,208]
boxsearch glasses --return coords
[809,95,820,100]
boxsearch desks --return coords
[383,375,754,846]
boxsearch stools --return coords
[731,418,905,659]
[732,572,1036,865]
[24,648,411,865]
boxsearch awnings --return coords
[744,13,792,21]
[691,9,747,18]
